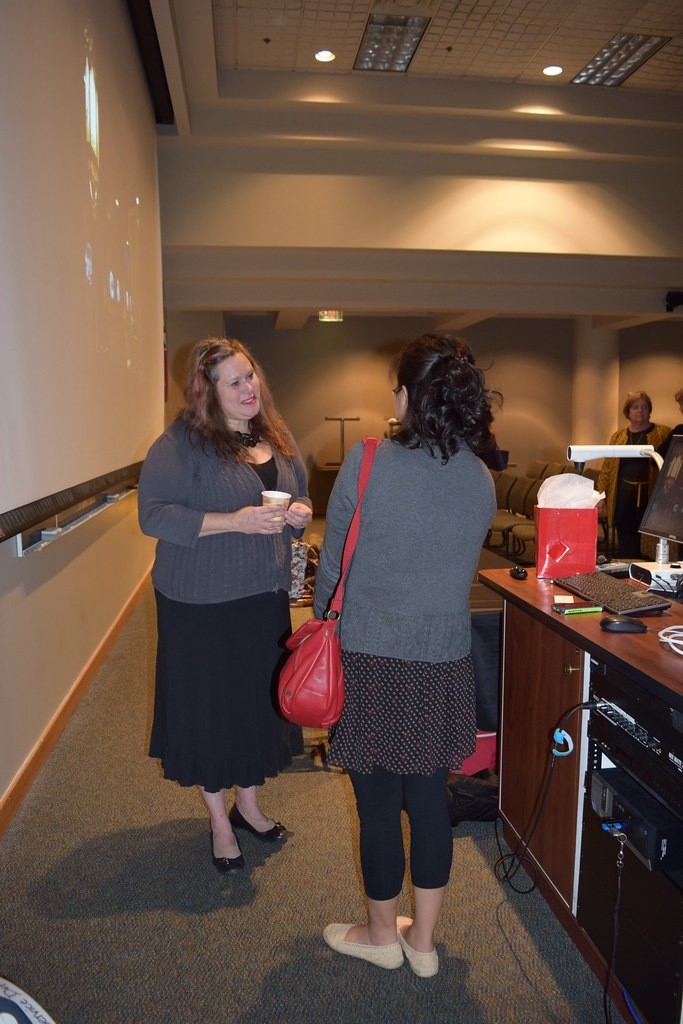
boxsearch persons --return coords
[312,331,498,978]
[597,390,683,562]
[138,336,312,875]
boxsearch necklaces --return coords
[233,430,261,448]
[630,427,644,445]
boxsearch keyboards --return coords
[552,570,672,614]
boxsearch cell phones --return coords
[551,600,604,613]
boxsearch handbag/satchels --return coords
[277,617,345,728]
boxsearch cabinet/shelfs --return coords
[477,563,683,1024]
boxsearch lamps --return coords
[318,310,344,322]
[568,444,683,586]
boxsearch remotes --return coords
[509,566,527,579]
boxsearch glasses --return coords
[392,385,405,397]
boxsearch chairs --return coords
[486,458,610,569]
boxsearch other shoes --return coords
[229,802,287,842]
[322,922,404,969]
[212,822,244,875]
[396,916,439,978]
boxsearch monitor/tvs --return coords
[635,434,683,544]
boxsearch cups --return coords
[261,490,292,534]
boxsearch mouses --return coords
[599,615,648,633]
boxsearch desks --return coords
[315,464,342,471]
